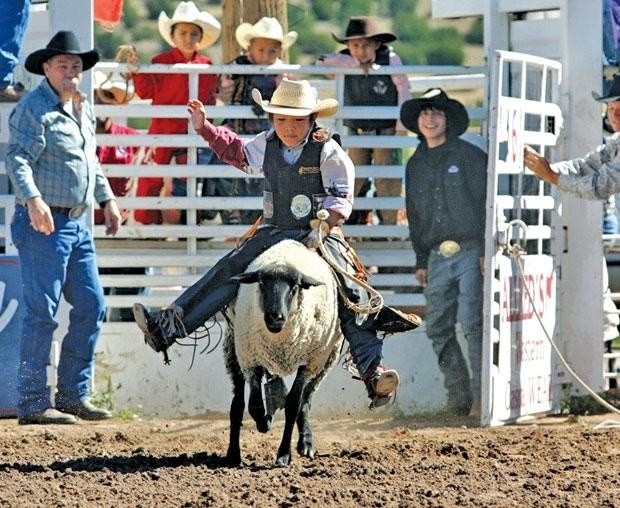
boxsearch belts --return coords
[25,202,86,220]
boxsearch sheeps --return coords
[224,240,346,468]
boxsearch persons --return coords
[93,69,164,226]
[1,0,32,101]
[217,16,300,225]
[127,1,224,229]
[398,85,488,422]
[314,14,411,240]
[525,71,620,201]
[3,31,123,423]
[133,76,422,413]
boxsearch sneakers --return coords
[55,393,113,421]
[0,80,27,104]
[467,398,482,422]
[18,405,78,425]
[132,301,175,354]
[432,396,472,421]
[363,368,399,409]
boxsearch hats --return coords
[24,30,100,75]
[330,16,397,44]
[591,73,620,103]
[234,16,299,51]
[399,87,470,138]
[157,1,221,50]
[251,76,340,119]
[94,70,133,106]
